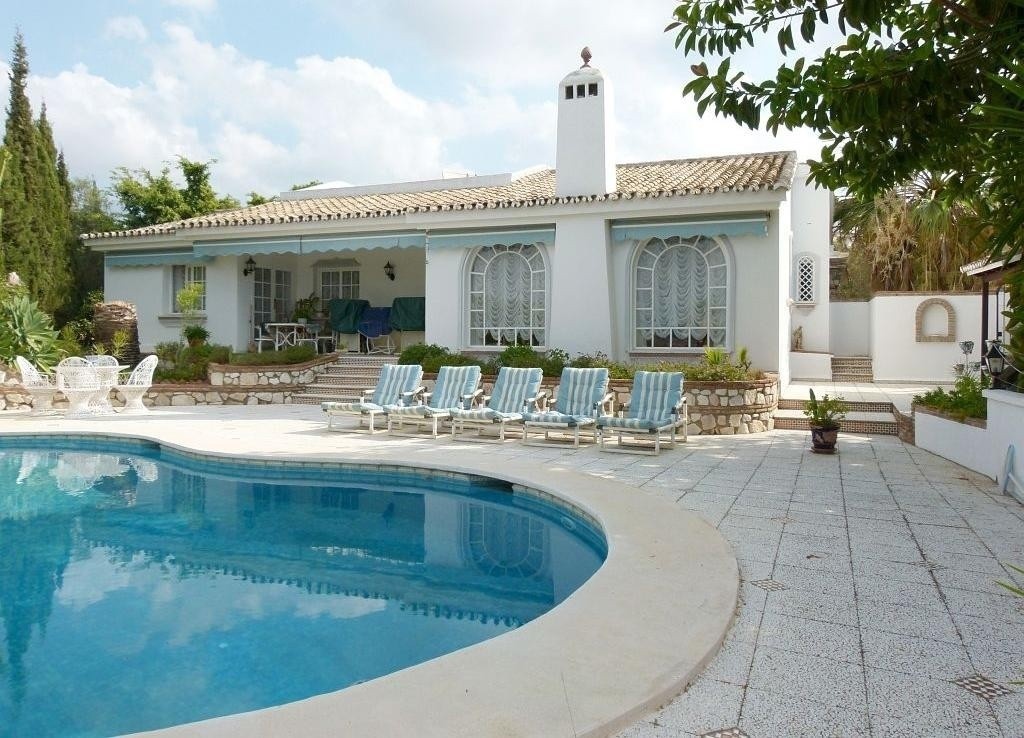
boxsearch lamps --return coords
[981,346,1007,389]
[243,256,256,276]
[384,261,395,280]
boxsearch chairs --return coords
[384,366,483,440]
[16,356,60,416]
[450,366,547,443]
[110,355,158,414]
[523,366,616,447]
[322,364,423,434]
[86,355,118,415]
[592,370,687,454]
[55,356,97,419]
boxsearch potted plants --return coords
[803,388,847,451]
[184,325,208,347]
[295,308,309,324]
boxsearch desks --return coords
[49,365,130,417]
[266,322,319,354]
